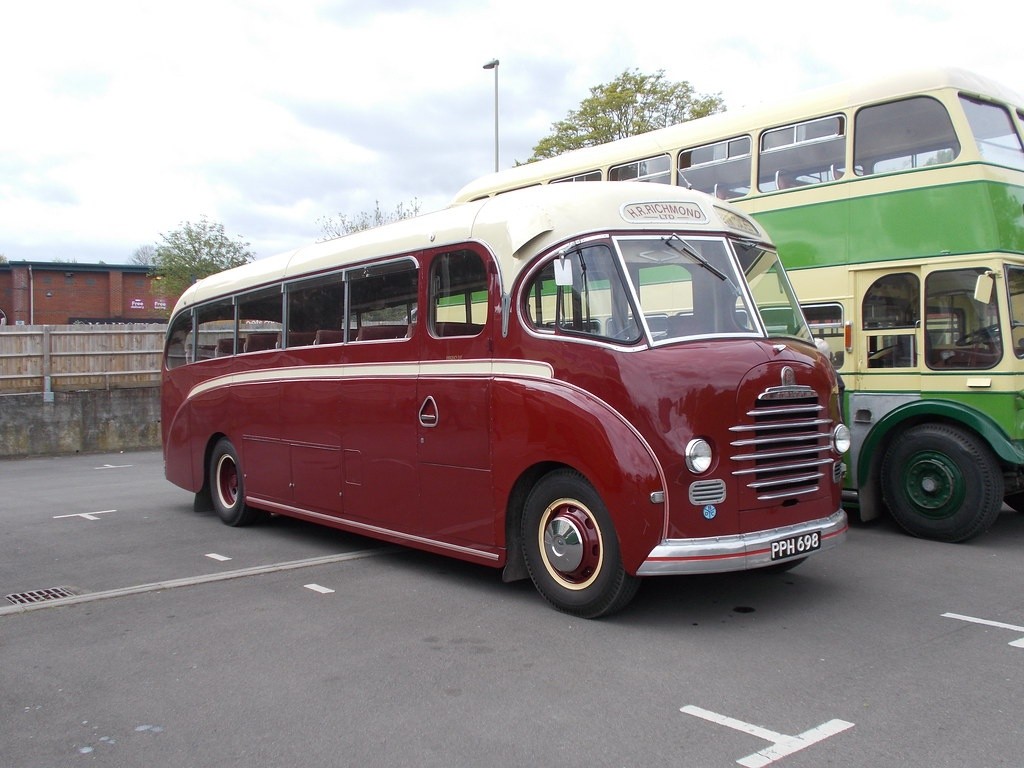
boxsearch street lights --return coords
[482,57,500,173]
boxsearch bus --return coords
[434,60,1024,544]
[158,178,851,620]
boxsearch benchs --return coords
[185,323,485,362]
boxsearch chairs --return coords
[711,164,843,202]
